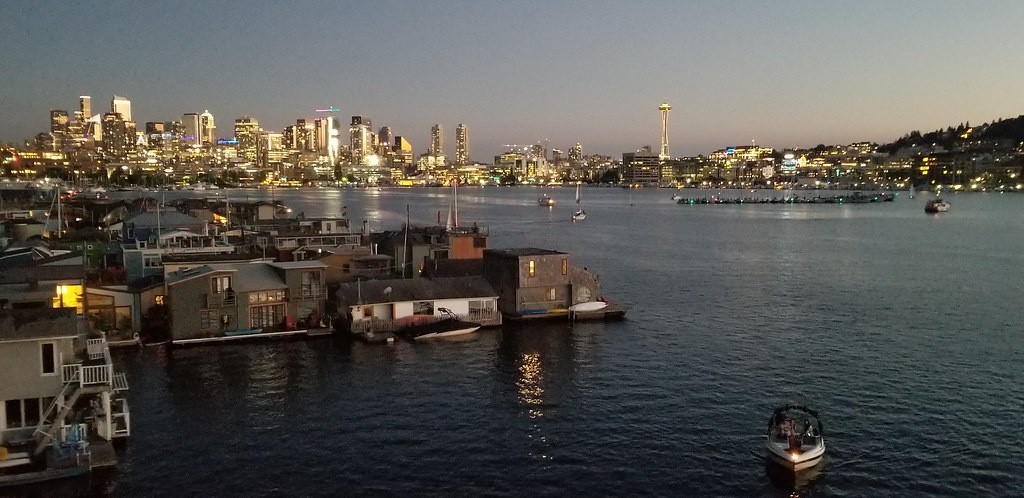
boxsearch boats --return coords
[765,404,826,471]
[925,199,951,212]
[538,194,554,206]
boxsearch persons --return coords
[802,419,814,437]
[789,419,797,436]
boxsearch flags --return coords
[446,191,456,231]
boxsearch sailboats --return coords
[446,180,467,233]
[572,184,586,220]
